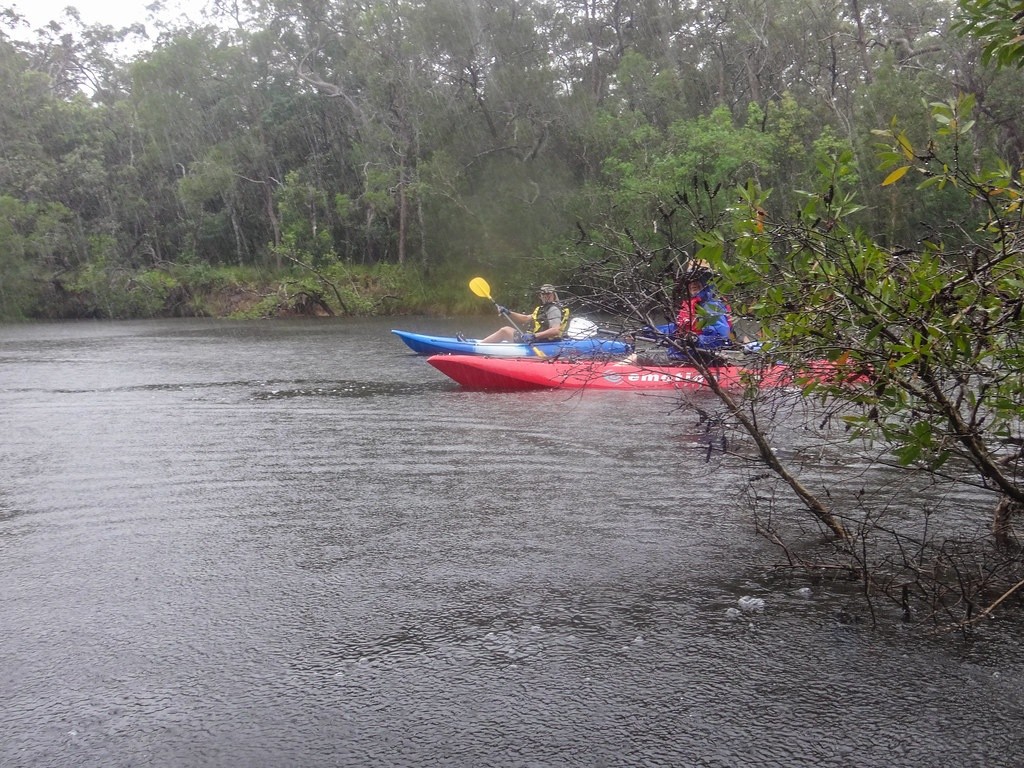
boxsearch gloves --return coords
[673,338,694,354]
[621,329,644,344]
[521,334,536,346]
[497,306,509,316]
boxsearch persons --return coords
[614,258,733,367]
[455,284,563,345]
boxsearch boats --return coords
[391,329,632,360]
[425,354,903,391]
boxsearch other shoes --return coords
[456,331,466,342]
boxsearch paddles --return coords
[468,276,546,358]
[566,317,771,363]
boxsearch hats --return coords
[540,283,555,294]
[686,258,713,275]
